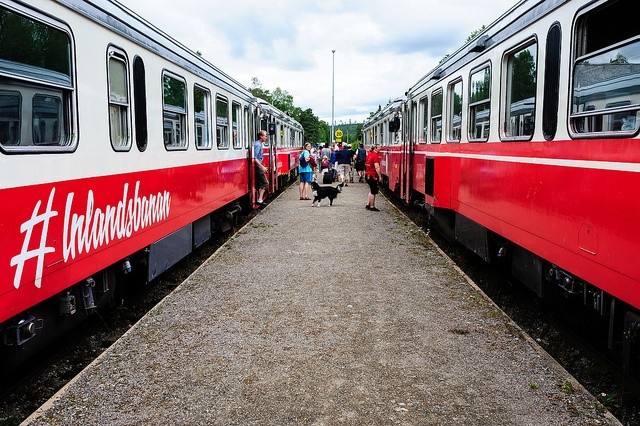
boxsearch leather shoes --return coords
[257,202,267,205]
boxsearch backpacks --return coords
[299,153,308,167]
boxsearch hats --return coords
[342,143,347,146]
[347,144,352,147]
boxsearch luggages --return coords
[323,168,333,184]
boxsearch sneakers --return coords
[362,181,364,183]
[370,207,380,211]
[366,205,369,209]
[359,176,362,182]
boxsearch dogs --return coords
[308,181,341,207]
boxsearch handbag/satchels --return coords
[354,162,357,169]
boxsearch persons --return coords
[321,156,330,176]
[311,145,322,172]
[332,140,353,186]
[322,144,331,159]
[354,142,366,182]
[253,130,270,206]
[366,143,382,211]
[299,142,313,201]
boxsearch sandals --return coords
[305,197,311,200]
[300,197,305,200]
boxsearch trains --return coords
[361,0,640,350]
[0,0,304,346]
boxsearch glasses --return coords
[306,143,308,146]
[376,144,379,149]
[265,136,267,137]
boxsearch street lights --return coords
[332,50,336,146]
